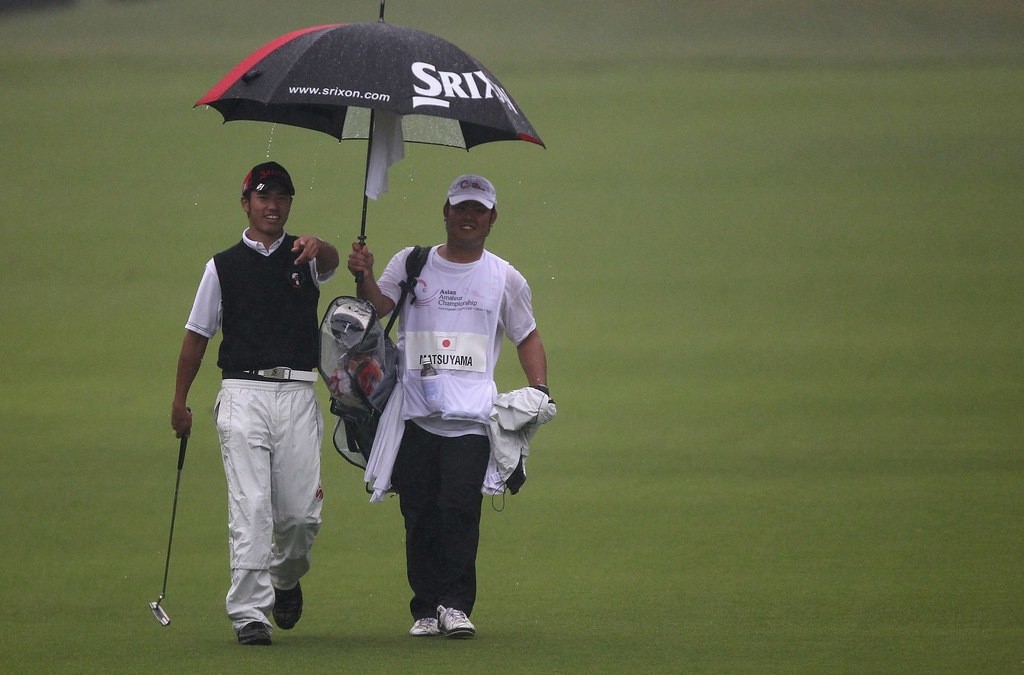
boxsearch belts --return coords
[243,366,320,383]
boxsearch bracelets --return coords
[529,384,549,396]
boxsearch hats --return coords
[242,162,295,198]
[446,174,497,209]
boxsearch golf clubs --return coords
[148,407,192,625]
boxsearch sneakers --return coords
[436,604,476,637]
[237,622,273,645]
[273,581,303,630]
[409,616,441,635]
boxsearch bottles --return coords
[420,357,438,402]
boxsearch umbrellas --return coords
[193,0,547,285]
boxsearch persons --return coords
[171,162,340,645]
[347,173,548,640]
